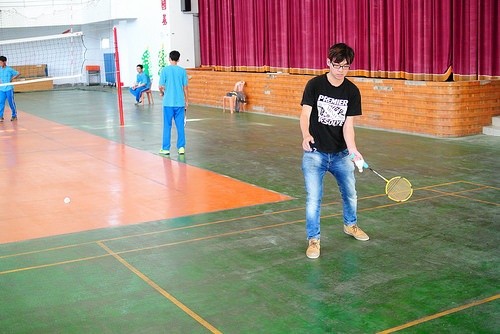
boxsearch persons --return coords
[159,50,188,154]
[129,64,151,104]
[300,43,370,259]
[0,56,20,122]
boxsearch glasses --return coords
[331,61,351,70]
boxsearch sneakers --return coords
[306,237,320,259]
[344,223,369,241]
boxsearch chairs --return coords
[223,81,245,113]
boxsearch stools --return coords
[141,90,153,105]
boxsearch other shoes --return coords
[178,147,184,154]
[134,99,142,105]
[160,149,170,155]
[0,118,4,122]
[11,116,17,121]
